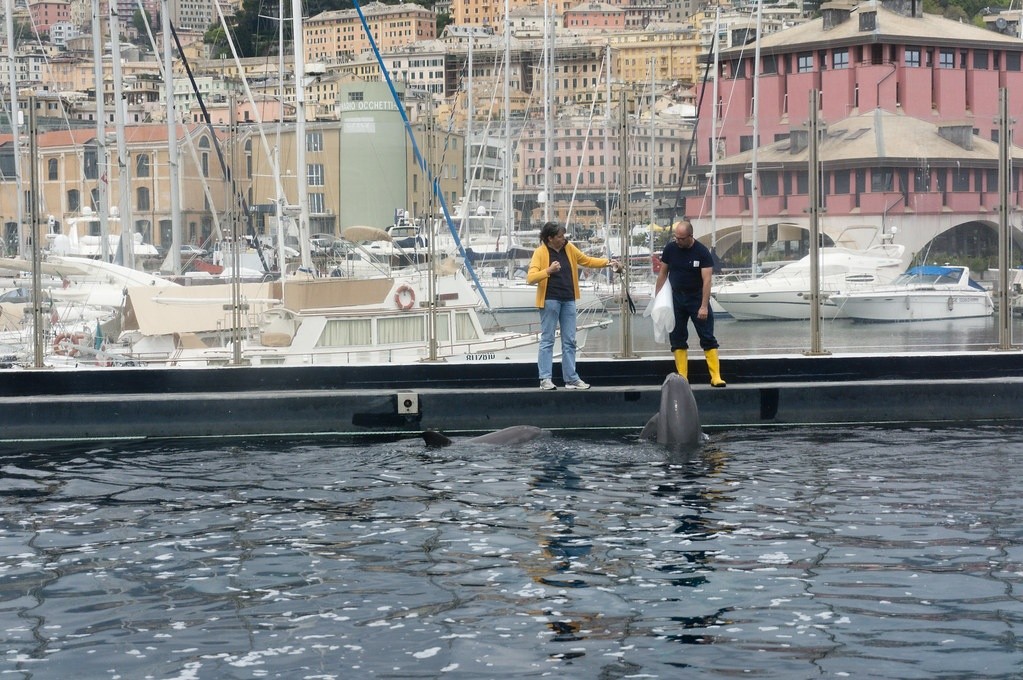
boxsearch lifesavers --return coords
[395,285,414,310]
[53,332,79,356]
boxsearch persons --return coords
[655,221,726,387]
[527,222,618,390]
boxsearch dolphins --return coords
[639,372,709,445]
[422,423,552,448]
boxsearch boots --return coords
[704,348,727,387]
[672,349,688,385]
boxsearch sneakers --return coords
[540,378,557,390]
[565,378,590,389]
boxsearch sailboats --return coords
[2,1,1023,355]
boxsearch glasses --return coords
[675,233,694,241]
